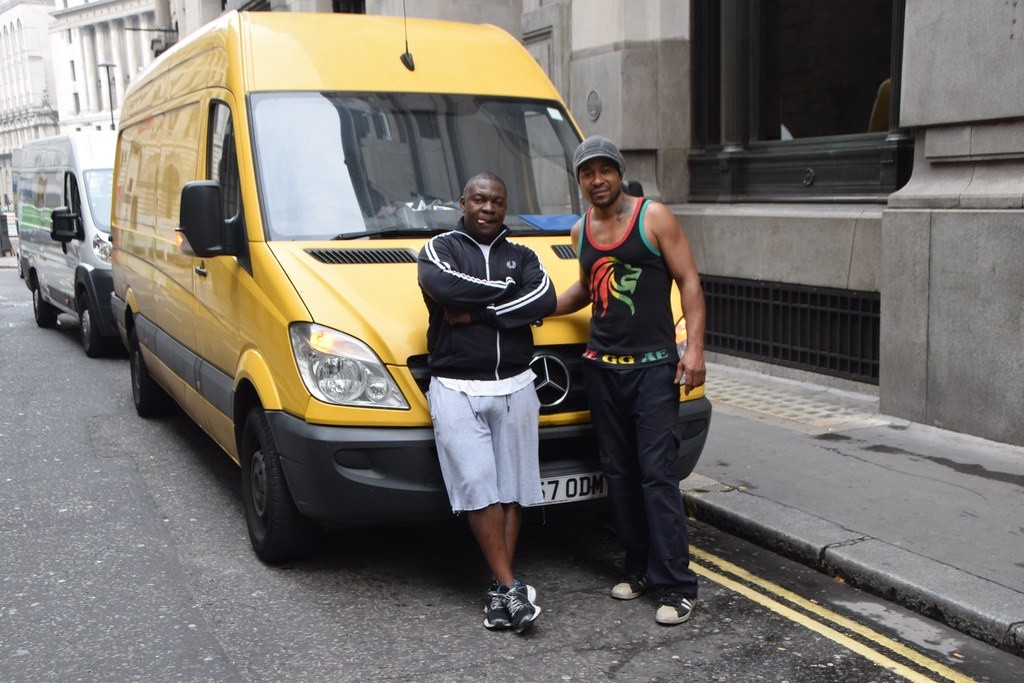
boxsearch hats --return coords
[572,135,625,183]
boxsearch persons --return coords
[417,170,557,634]
[550,135,708,625]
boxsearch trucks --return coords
[109,10,714,567]
[11,129,120,358]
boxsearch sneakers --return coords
[492,583,541,633]
[610,565,651,600]
[482,580,513,630]
[656,592,696,625]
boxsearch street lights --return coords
[98,63,118,130]
